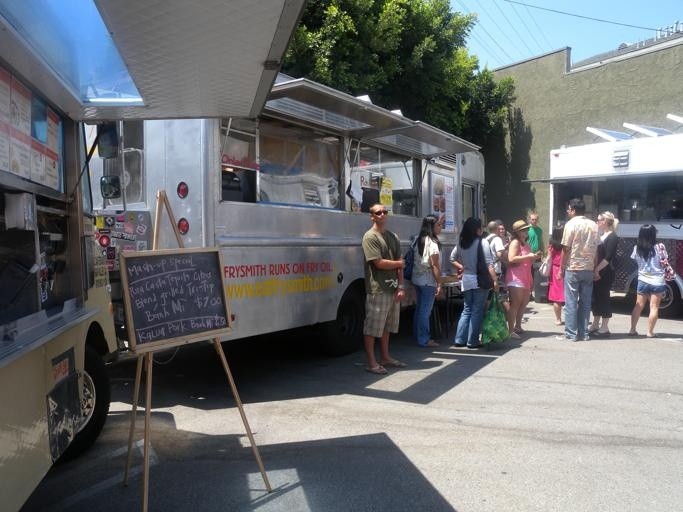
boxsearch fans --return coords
[118,247,235,351]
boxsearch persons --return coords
[586,211,619,338]
[482,220,511,318]
[627,224,669,338]
[555,198,599,342]
[504,218,542,339]
[360,203,408,375]
[546,224,566,326]
[410,213,447,347]
[525,213,543,301]
[496,224,511,250]
[446,217,501,349]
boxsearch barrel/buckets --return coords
[627,332,637,336]
[645,335,657,338]
[366,365,388,374]
[380,358,408,367]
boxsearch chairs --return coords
[509,332,520,339]
[555,334,566,340]
[576,336,587,340]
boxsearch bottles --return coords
[511,220,531,234]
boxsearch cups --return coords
[375,210,388,216]
[596,219,603,222]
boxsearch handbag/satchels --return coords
[398,285,405,291]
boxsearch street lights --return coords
[655,242,676,281]
[476,236,492,288]
[538,248,552,277]
[404,236,419,280]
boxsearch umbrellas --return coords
[468,345,482,348]
[455,343,465,347]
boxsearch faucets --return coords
[427,340,439,347]
[587,328,609,338]
